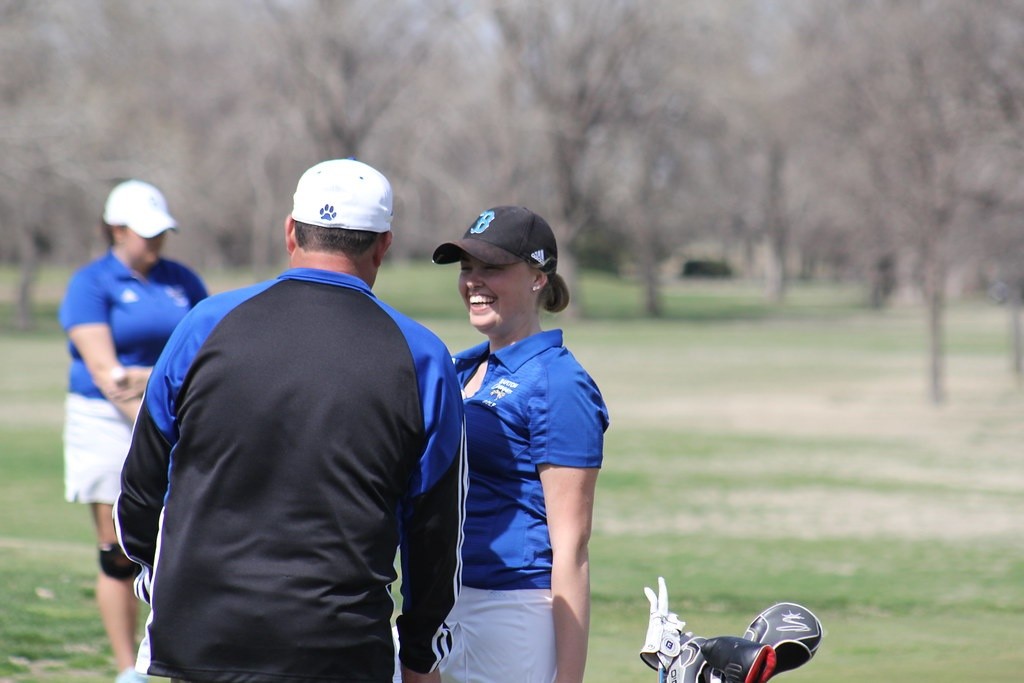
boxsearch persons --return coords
[109,156,470,683]
[430,205,610,683]
[57,180,210,683]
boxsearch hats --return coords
[293,158,392,233]
[434,206,556,267]
[104,179,179,237]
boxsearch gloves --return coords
[640,578,692,670]
